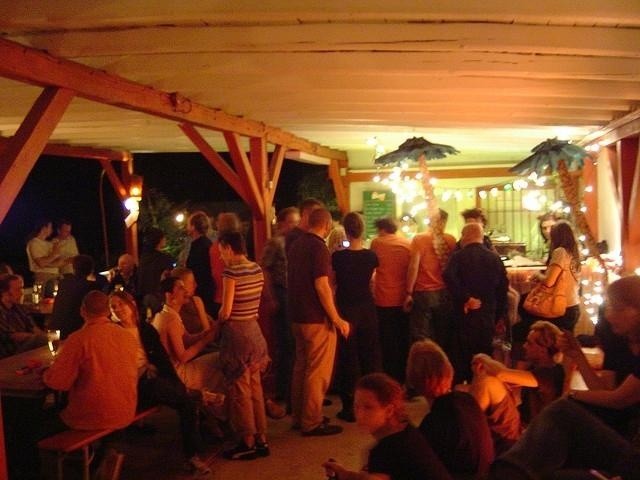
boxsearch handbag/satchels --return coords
[523,263,567,319]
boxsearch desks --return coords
[0,341,70,480]
[500,255,548,343]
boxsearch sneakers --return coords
[222,441,271,459]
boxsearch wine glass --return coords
[47,330,61,357]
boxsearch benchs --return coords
[37,396,169,479]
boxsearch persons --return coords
[0,197,640,480]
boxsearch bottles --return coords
[32,283,41,305]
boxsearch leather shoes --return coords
[293,399,355,435]
